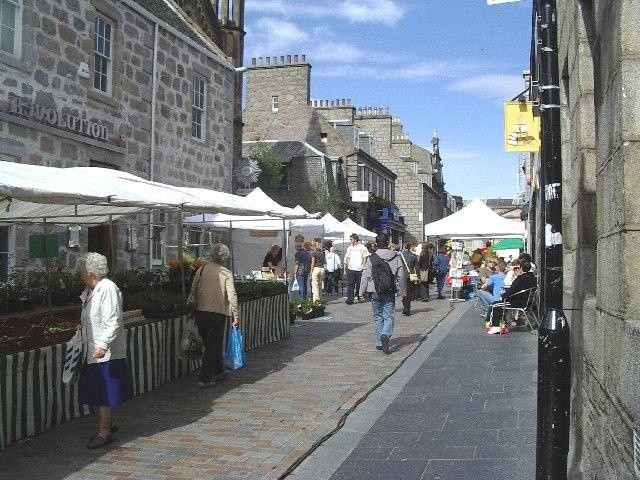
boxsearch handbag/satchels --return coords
[369,252,397,297]
[333,268,342,282]
[420,269,428,282]
[61,328,83,386]
[186,282,201,313]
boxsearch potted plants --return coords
[0,252,84,313]
[234,279,286,302]
[109,253,208,296]
[289,298,327,324]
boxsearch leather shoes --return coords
[87,433,111,448]
[110,424,118,433]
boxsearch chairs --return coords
[472,285,540,336]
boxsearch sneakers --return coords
[486,326,501,335]
[381,335,389,352]
[215,370,230,381]
[199,380,215,389]
[376,345,382,349]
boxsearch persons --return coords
[186,242,240,389]
[74,251,134,449]
[358,232,408,356]
[261,233,538,334]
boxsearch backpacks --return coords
[409,272,419,283]
